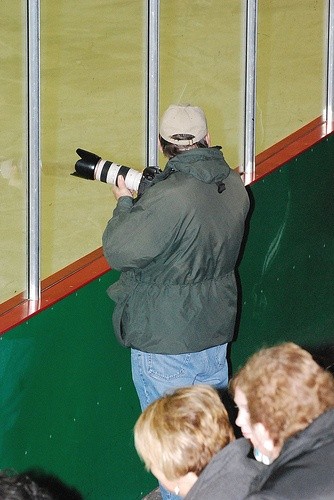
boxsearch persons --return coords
[229,341,334,500]
[132,384,269,500]
[101,103,250,500]
[0,467,84,500]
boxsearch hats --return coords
[159,104,207,145]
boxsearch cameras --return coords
[70,148,162,196]
